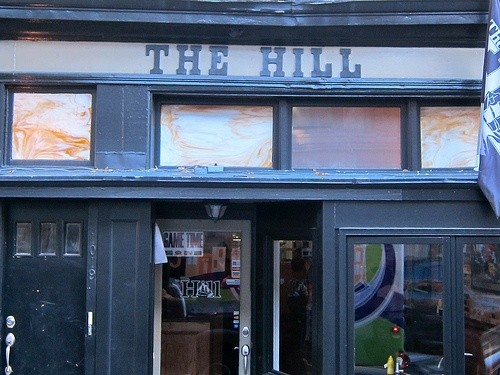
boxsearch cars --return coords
[403,256,500,375]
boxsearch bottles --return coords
[387,355,395,375]
[394,349,407,375]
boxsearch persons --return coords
[3,284,30,367]
[26,259,63,304]
[463,243,499,282]
[288,257,311,326]
[162,263,184,321]
